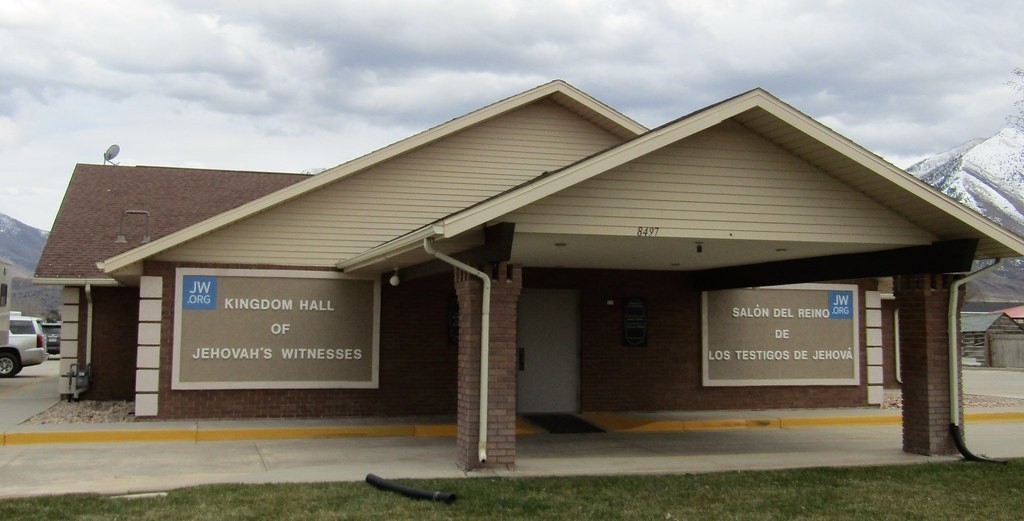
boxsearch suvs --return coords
[0,310,48,378]
[41,323,61,354]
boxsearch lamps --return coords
[390,267,401,286]
